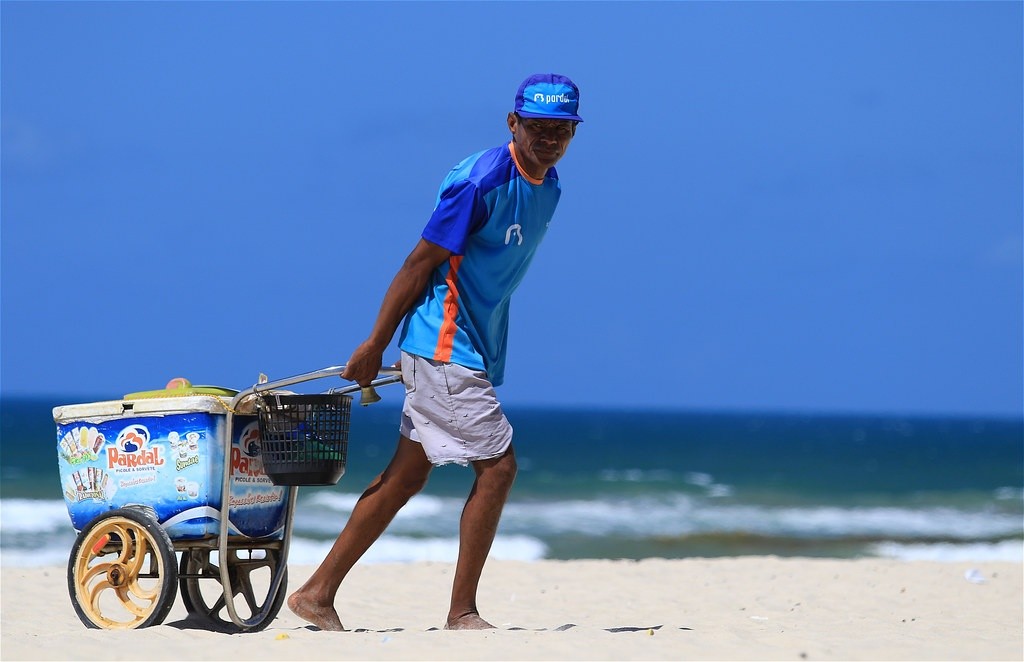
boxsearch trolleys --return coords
[52,363,404,634]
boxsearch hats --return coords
[514,73,584,123]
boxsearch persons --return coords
[287,74,584,634]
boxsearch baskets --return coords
[256,393,354,486]
